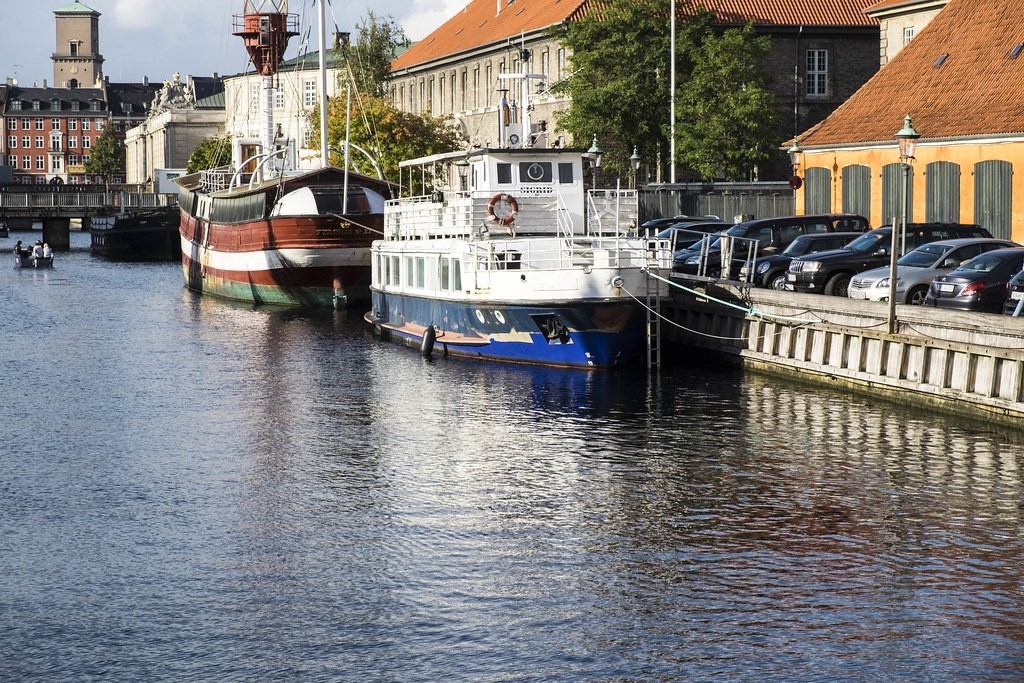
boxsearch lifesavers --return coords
[487,193,520,225]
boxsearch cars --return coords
[1000,269,1024,319]
[634,212,878,289]
[847,236,1024,307]
[737,231,879,291]
[922,247,1024,314]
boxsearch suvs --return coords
[783,222,999,298]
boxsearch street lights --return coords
[893,111,922,258]
[586,133,606,197]
[628,145,643,189]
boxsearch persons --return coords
[143,175,151,188]
[32,240,52,260]
[14,241,25,257]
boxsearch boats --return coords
[168,1,412,310]
[14,248,55,269]
[87,201,182,258]
[364,29,674,373]
